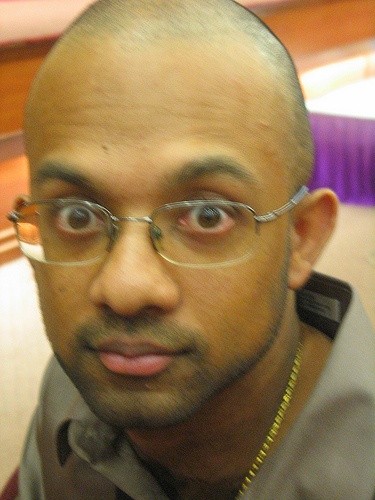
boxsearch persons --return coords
[0,0,375,500]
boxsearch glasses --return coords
[8,184,309,266]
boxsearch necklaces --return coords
[233,324,306,499]
